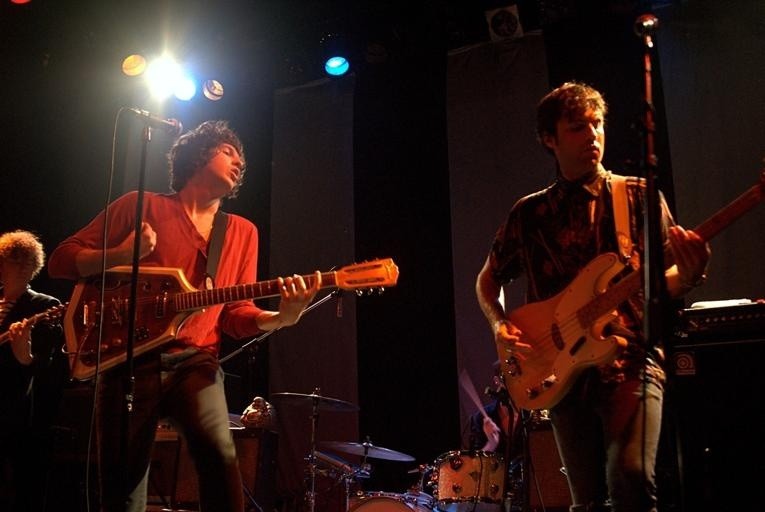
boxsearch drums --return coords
[432,450,507,512]
[297,449,370,512]
[347,491,431,512]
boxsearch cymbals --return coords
[321,440,415,462]
[268,392,360,413]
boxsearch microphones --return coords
[131,107,183,137]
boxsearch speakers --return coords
[656,303,764,510]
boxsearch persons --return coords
[462,364,529,512]
[475,81,713,511]
[0,228,65,510]
[47,118,323,510]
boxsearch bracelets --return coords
[684,272,708,288]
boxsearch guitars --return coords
[495,179,764,416]
[0,300,70,345]
[62,256,399,383]
